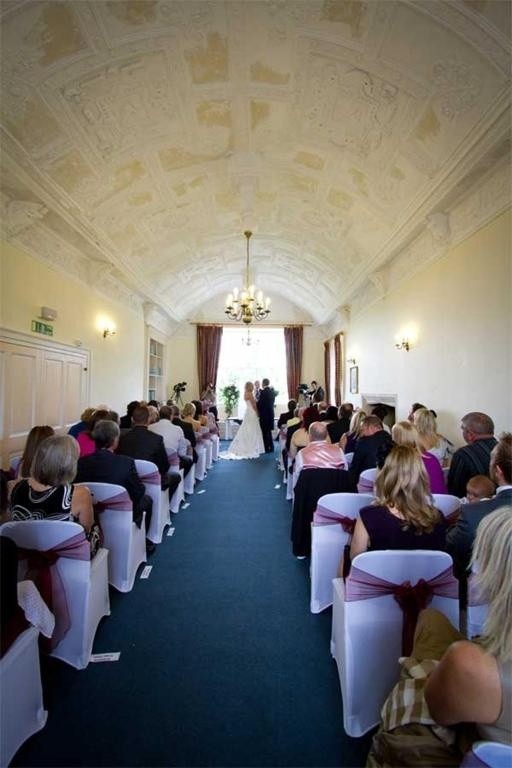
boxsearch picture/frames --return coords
[350,366,358,394]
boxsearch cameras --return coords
[210,386,214,389]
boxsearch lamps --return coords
[96,311,115,339]
[395,324,415,352]
[225,231,271,346]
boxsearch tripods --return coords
[175,392,184,408]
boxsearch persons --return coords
[218,381,266,460]
[449,411,502,499]
[276,382,452,506]
[256,379,274,454]
[254,381,262,402]
[15,425,55,479]
[447,433,511,588]
[67,398,216,504]
[424,504,512,767]
[339,443,448,583]
[6,433,103,560]
[200,382,216,402]
[73,419,155,558]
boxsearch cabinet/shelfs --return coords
[144,324,168,406]
[0,327,92,472]
